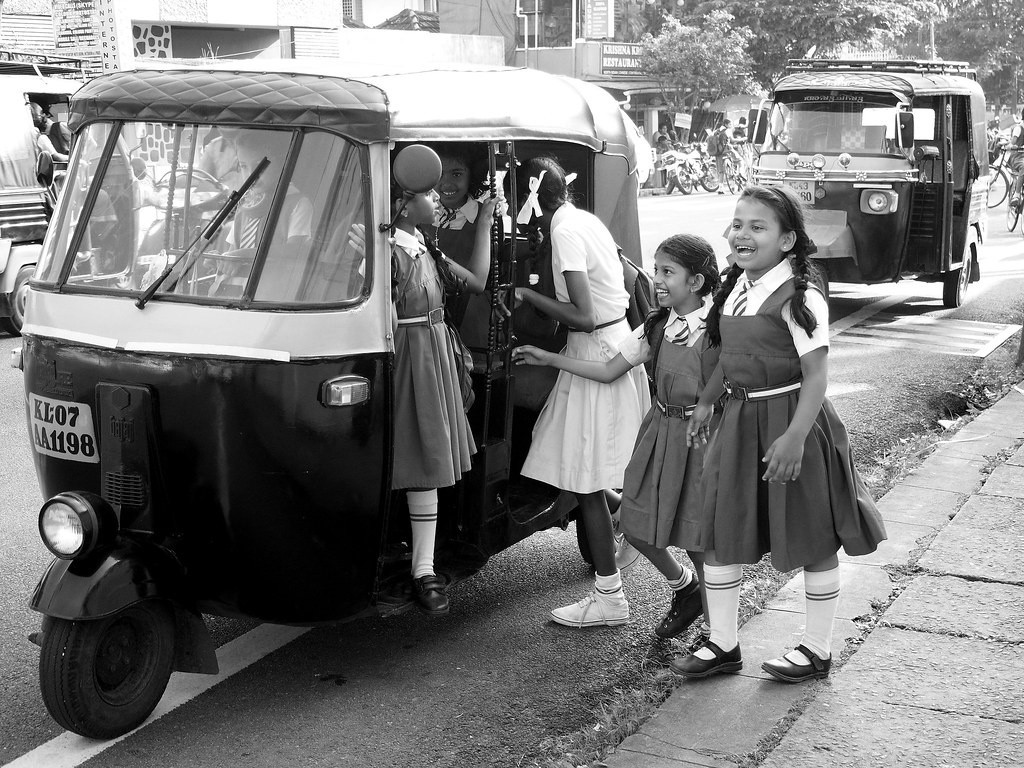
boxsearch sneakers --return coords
[655,575,704,638]
[549,594,630,627]
[615,536,642,574]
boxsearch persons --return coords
[31,100,755,652]
[666,184,890,682]
[988,107,1024,209]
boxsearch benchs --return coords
[892,138,970,214]
[463,238,568,410]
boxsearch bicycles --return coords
[721,140,753,195]
[987,140,1024,236]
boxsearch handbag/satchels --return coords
[617,242,660,330]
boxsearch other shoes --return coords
[1010,198,1018,204]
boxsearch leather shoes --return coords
[668,642,742,678]
[410,569,449,617]
[761,645,832,682]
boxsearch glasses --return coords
[35,113,46,120]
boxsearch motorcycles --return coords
[747,58,991,307]
[1,60,642,742]
[658,133,720,195]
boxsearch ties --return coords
[672,319,690,346]
[732,281,754,316]
[437,208,458,228]
[240,217,260,248]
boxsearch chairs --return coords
[840,125,888,154]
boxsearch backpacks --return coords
[707,130,726,155]
